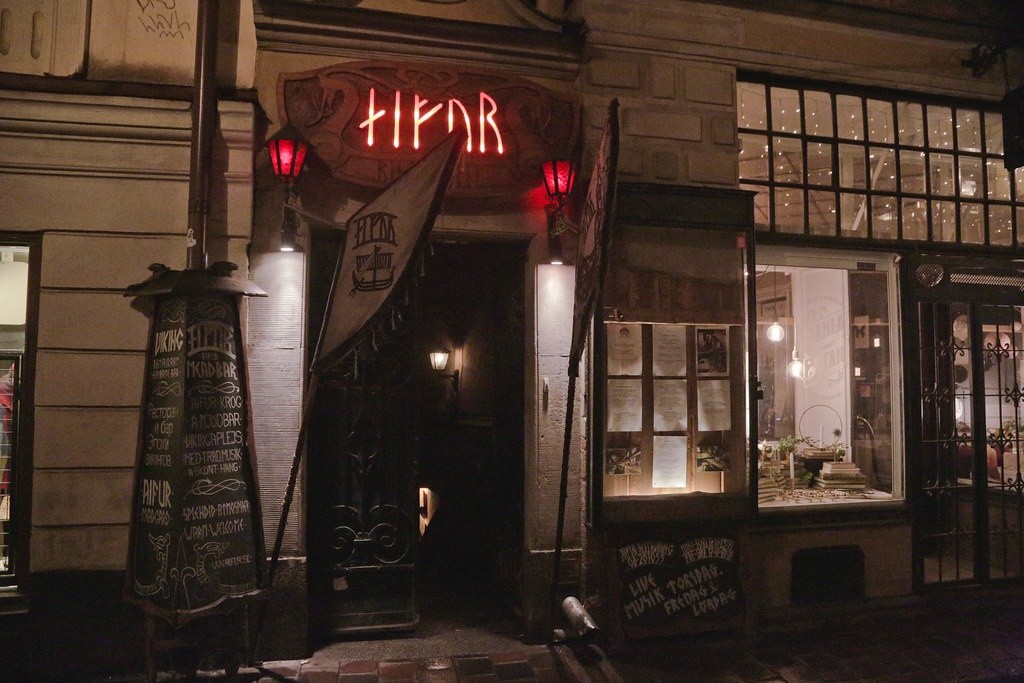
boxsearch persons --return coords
[0,361,15,507]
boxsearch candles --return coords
[820,425,824,449]
[790,452,794,479]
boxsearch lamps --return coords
[428,342,460,394]
[263,118,309,254]
[538,134,580,265]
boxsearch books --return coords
[801,447,840,459]
[813,461,868,490]
[758,476,786,504]
